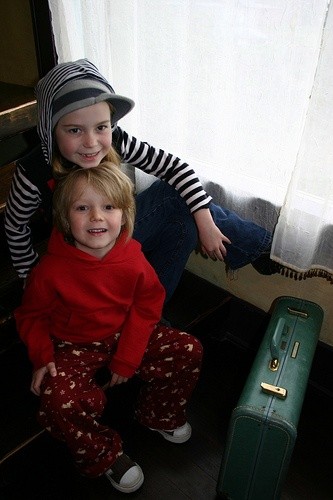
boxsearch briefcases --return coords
[214,294,325,499]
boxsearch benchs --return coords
[0,156,232,464]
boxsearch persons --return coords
[5,59,283,306]
[14,162,205,492]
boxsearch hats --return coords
[51,75,134,125]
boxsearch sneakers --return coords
[102,451,144,493]
[251,240,278,278]
[145,422,193,444]
